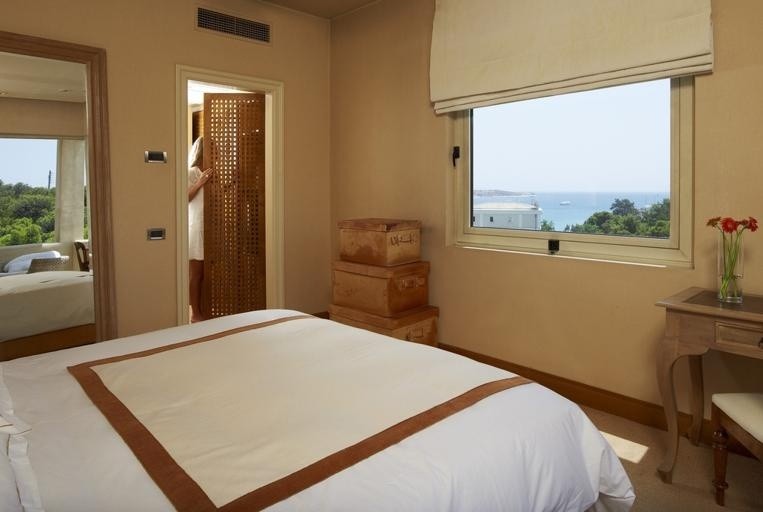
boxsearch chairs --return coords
[710,392,763,506]
[74,242,90,271]
[28,256,70,274]
[4,252,60,273]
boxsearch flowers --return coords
[705,217,758,293]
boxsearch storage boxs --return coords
[330,260,431,318]
[338,218,423,267]
[330,304,439,349]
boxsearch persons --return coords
[188,136,218,324]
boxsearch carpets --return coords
[576,399,763,512]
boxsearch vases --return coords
[719,235,743,303]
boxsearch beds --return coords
[0,309,635,512]
[0,272,94,342]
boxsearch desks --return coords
[655,287,763,484]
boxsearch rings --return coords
[205,174,208,177]
